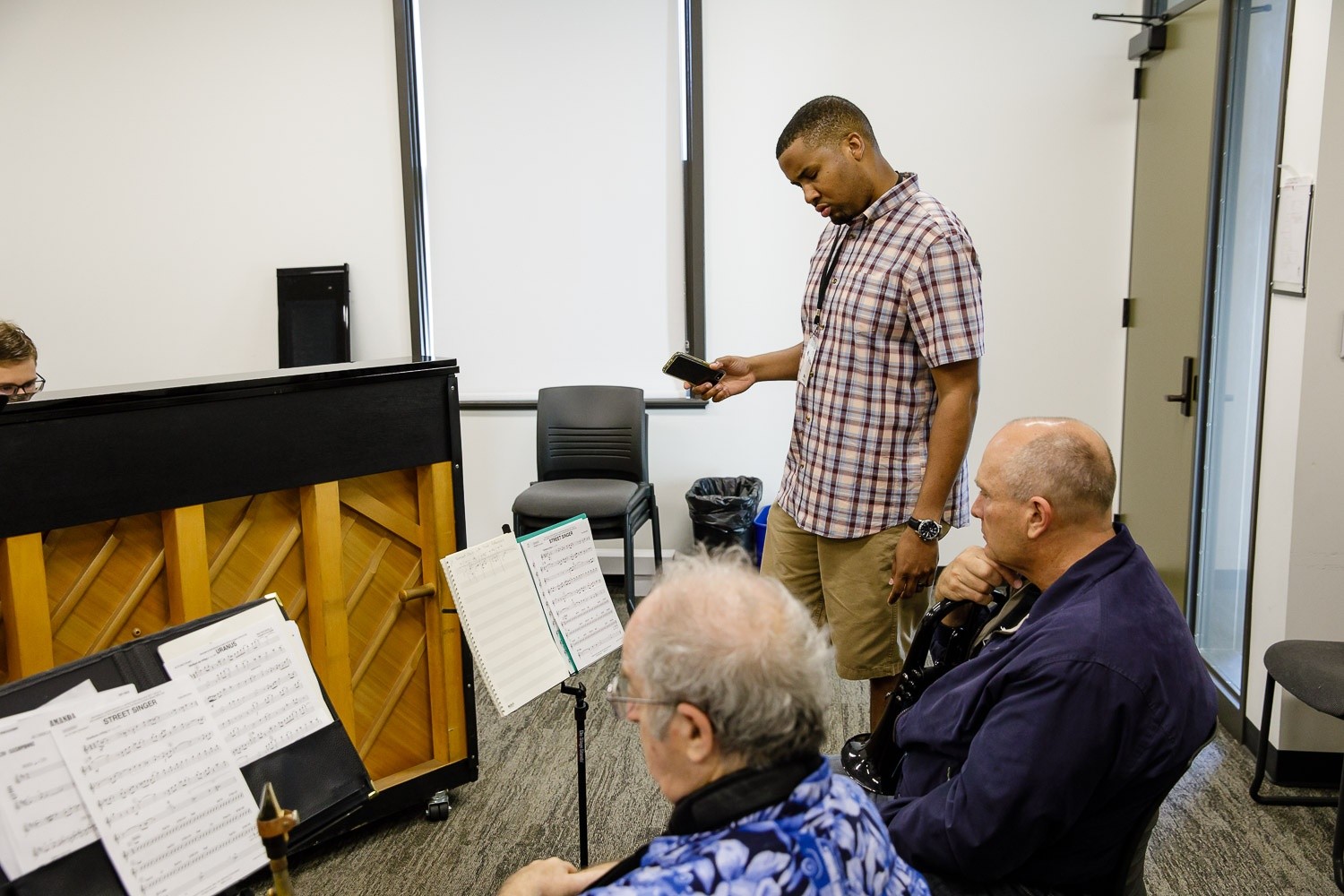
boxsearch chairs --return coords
[1114,716,1221,896]
[512,384,662,615]
[1250,639,1344,894]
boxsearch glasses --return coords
[604,675,677,720]
[0,373,45,398]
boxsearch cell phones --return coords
[662,352,727,387]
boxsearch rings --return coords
[918,581,926,585]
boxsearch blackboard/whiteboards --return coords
[0,0,712,411]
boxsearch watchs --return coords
[908,516,942,541]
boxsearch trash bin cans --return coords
[685,476,762,550]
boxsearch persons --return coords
[686,94,986,713]
[0,322,46,396]
[875,418,1216,896]
[493,567,929,896]
